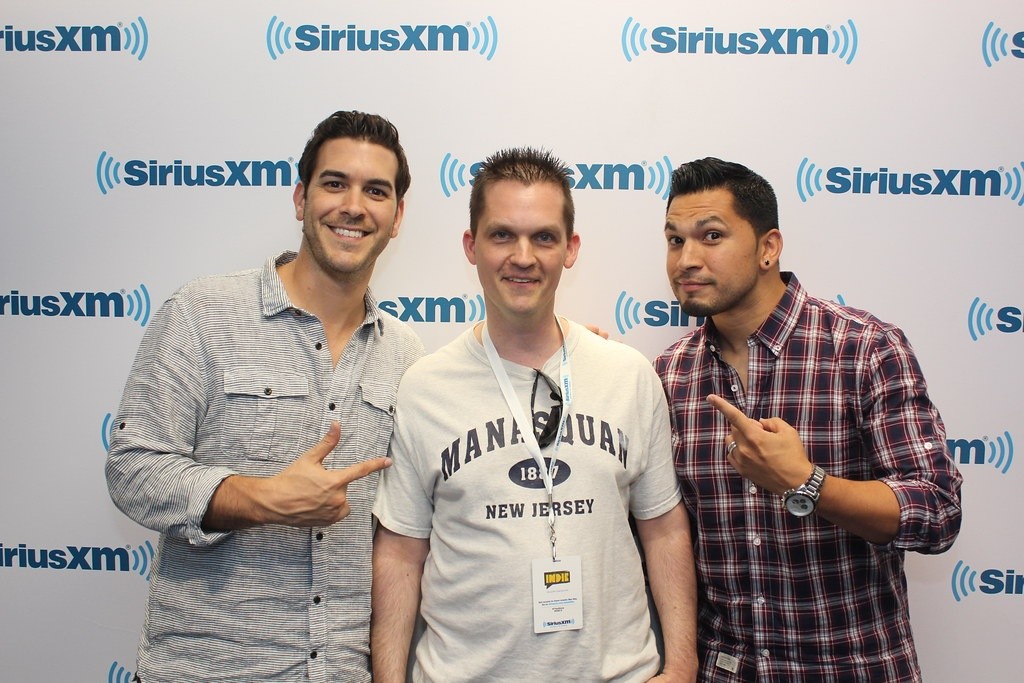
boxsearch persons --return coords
[105,110,623,683]
[630,157,963,683]
[370,149,701,683]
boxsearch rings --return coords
[728,441,736,455]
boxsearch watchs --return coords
[782,466,825,518]
